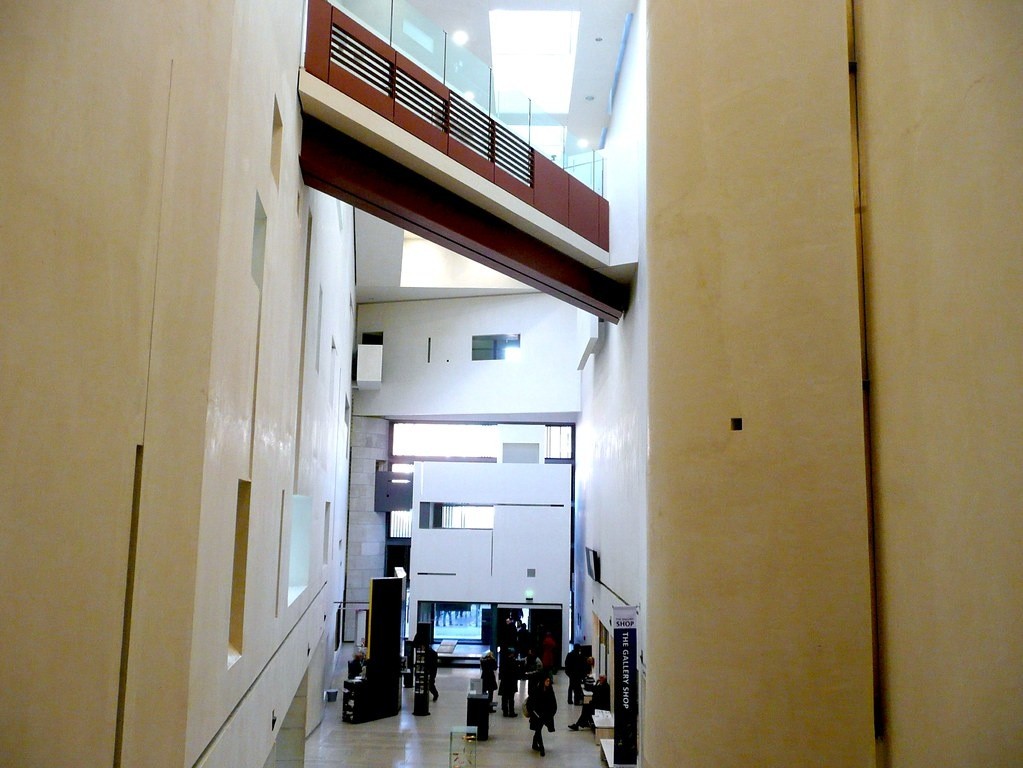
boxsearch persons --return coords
[481,650,496,713]
[501,617,557,666]
[528,676,557,756]
[428,644,438,702]
[499,647,526,717]
[565,643,594,705]
[568,675,610,730]
[348,653,363,680]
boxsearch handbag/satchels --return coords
[522,698,531,718]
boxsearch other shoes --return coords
[503,712,510,717]
[575,702,582,705]
[568,701,573,704]
[433,693,438,701]
[532,743,541,751]
[509,713,518,717]
[569,724,579,731]
[540,745,546,756]
[490,710,497,714]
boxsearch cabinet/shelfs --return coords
[466,693,493,741]
[413,644,432,716]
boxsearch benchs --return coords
[592,713,614,745]
[599,738,637,768]
[581,684,594,703]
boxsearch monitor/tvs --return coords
[585,546,600,581]
